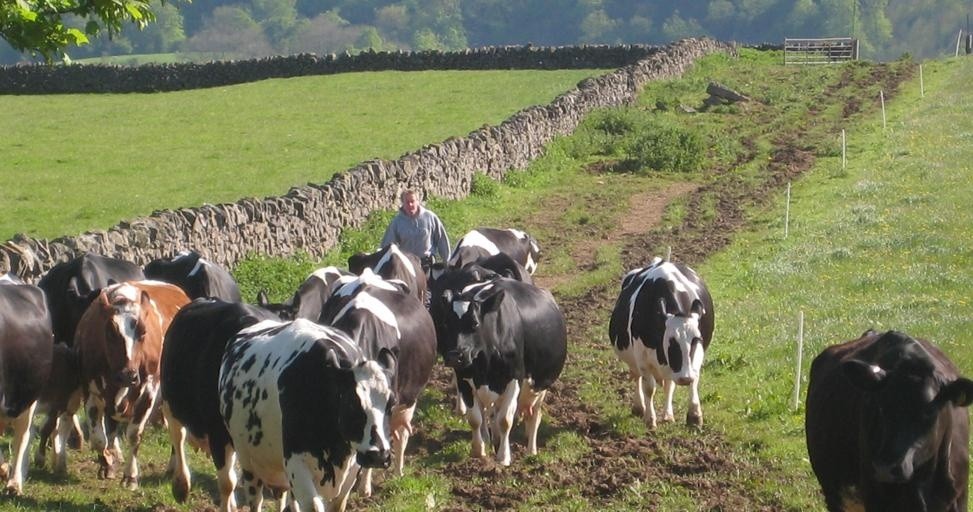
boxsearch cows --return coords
[804,327,973,511]
[0,226,568,512]
[608,255,715,432]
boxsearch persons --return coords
[380,187,451,269]
